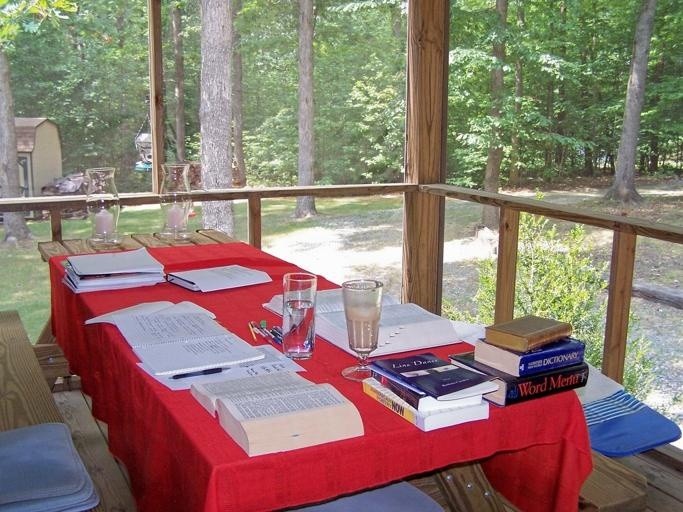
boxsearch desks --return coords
[37,227,592,512]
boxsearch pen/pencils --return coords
[167,367,231,379]
[249,320,283,344]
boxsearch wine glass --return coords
[340,277,383,381]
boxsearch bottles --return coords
[85,166,124,250]
[152,163,195,245]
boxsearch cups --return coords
[281,271,318,359]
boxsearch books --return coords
[60,246,168,295]
[485,314,572,352]
[445,352,589,408]
[257,282,465,362]
[185,367,365,459]
[357,350,500,434]
[109,312,266,375]
[472,336,587,378]
[81,298,216,327]
[166,275,200,292]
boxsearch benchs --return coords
[579,446,647,512]
[0,310,105,512]
